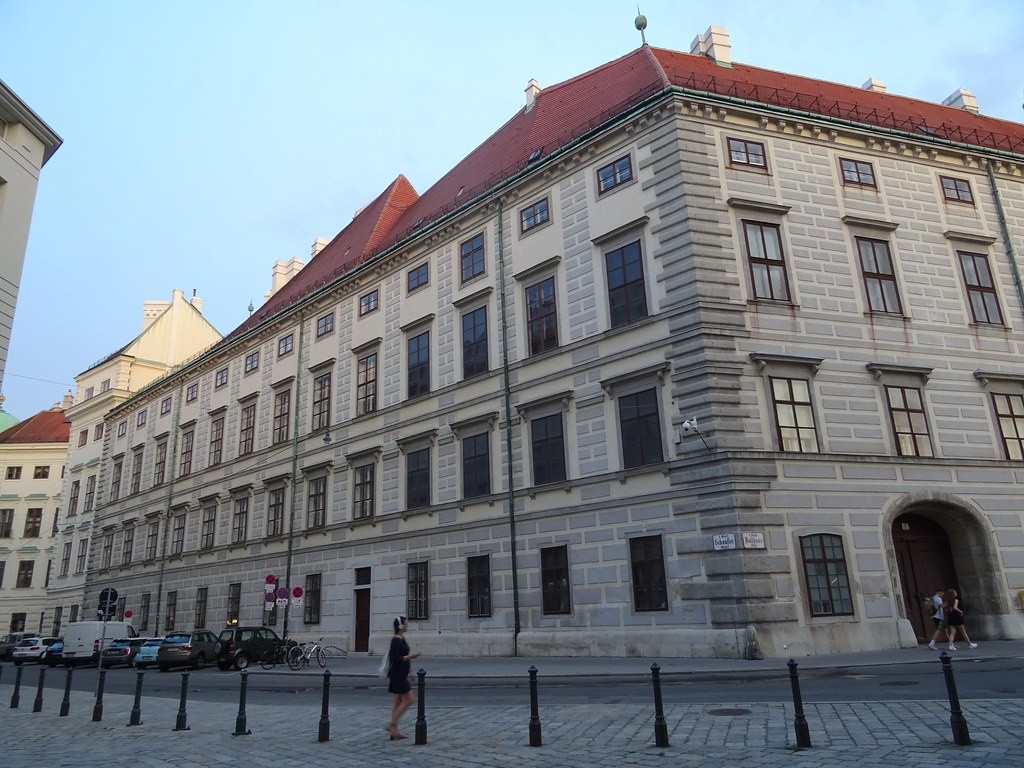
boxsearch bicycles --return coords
[261,638,296,670]
[287,638,327,671]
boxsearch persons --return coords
[384,616,421,740]
[929,589,977,651]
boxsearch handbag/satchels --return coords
[378,649,394,680]
[969,643,977,649]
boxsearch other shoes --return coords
[929,643,938,650]
[949,646,956,650]
[955,644,960,649]
[384,721,408,741]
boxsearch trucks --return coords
[61,622,143,668]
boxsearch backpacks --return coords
[924,596,940,616]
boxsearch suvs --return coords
[0,633,37,662]
[12,637,62,666]
[102,637,146,668]
[155,629,224,672]
[213,626,297,671]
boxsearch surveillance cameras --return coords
[683,423,690,431]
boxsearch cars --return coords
[134,637,164,669]
[46,643,87,667]
